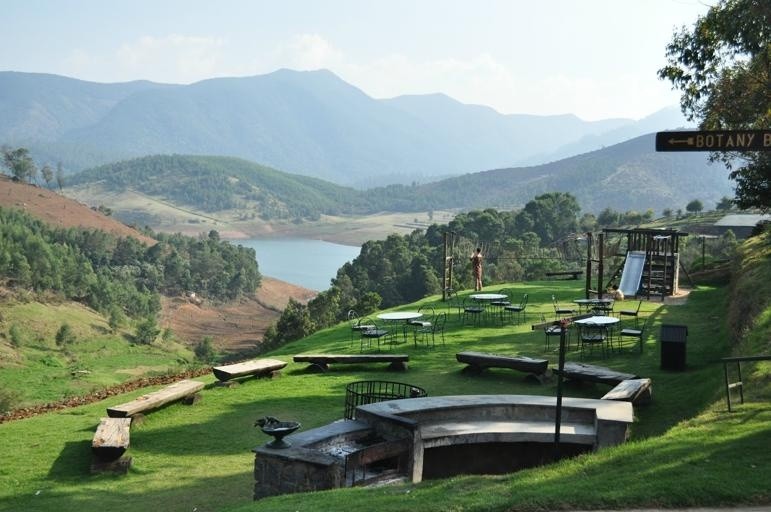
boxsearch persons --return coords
[470,247,484,290]
[608,285,625,302]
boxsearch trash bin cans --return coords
[660,325,688,372]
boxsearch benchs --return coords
[545,270,583,282]
[91,378,206,478]
[455,350,654,404]
[211,354,409,389]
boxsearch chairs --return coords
[443,285,647,361]
[346,305,447,354]
